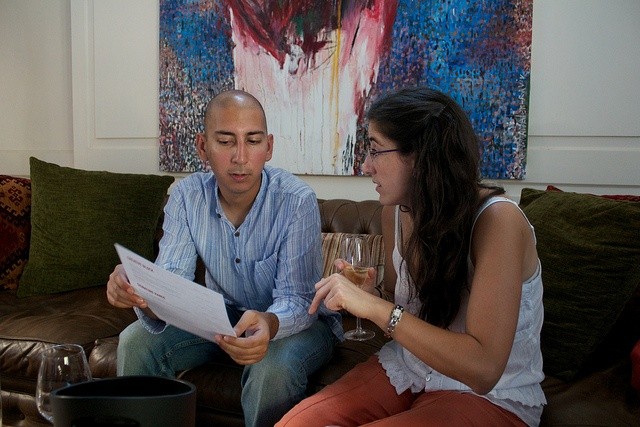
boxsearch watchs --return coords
[384,303,405,340]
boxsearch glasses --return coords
[367,147,399,160]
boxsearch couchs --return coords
[0,157,175,427]
[87,183,640,426]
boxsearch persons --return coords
[268,88,546,426]
[106,89,345,426]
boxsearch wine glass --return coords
[340,236,376,341]
[35,345,93,425]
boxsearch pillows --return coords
[0,176,33,291]
[518,188,640,385]
[15,157,176,297]
[546,186,639,205]
[322,232,384,292]
